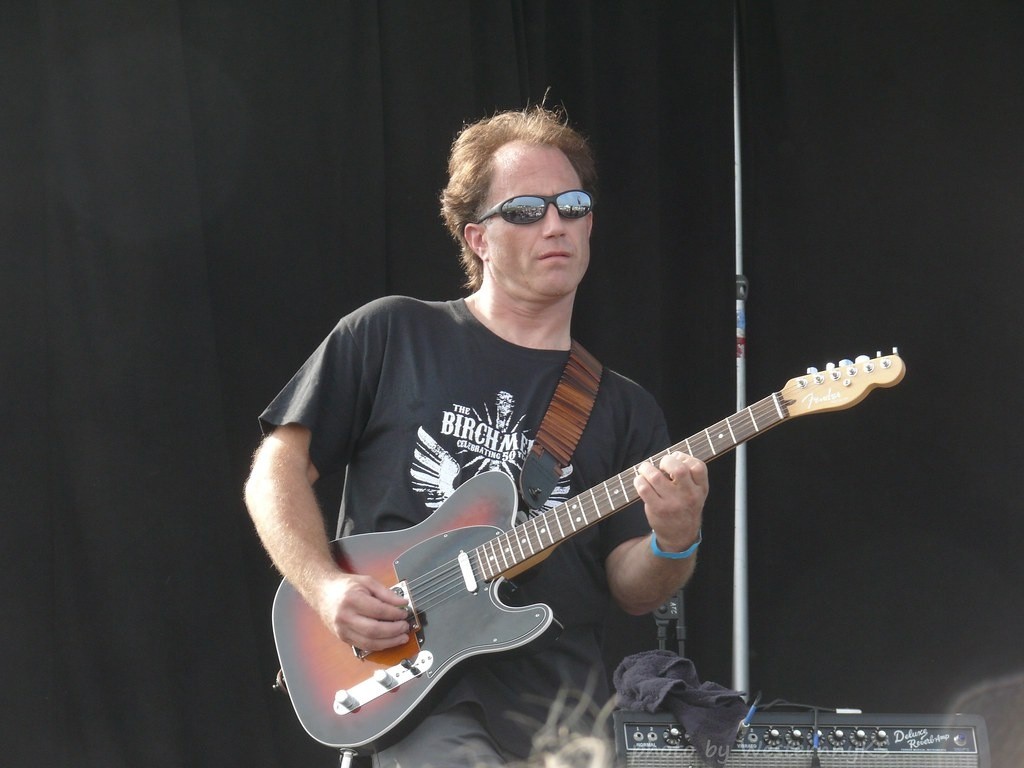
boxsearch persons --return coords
[241,87,710,767]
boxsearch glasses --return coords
[476,190,597,224]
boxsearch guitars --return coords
[270,344,909,755]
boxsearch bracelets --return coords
[651,530,702,560]
